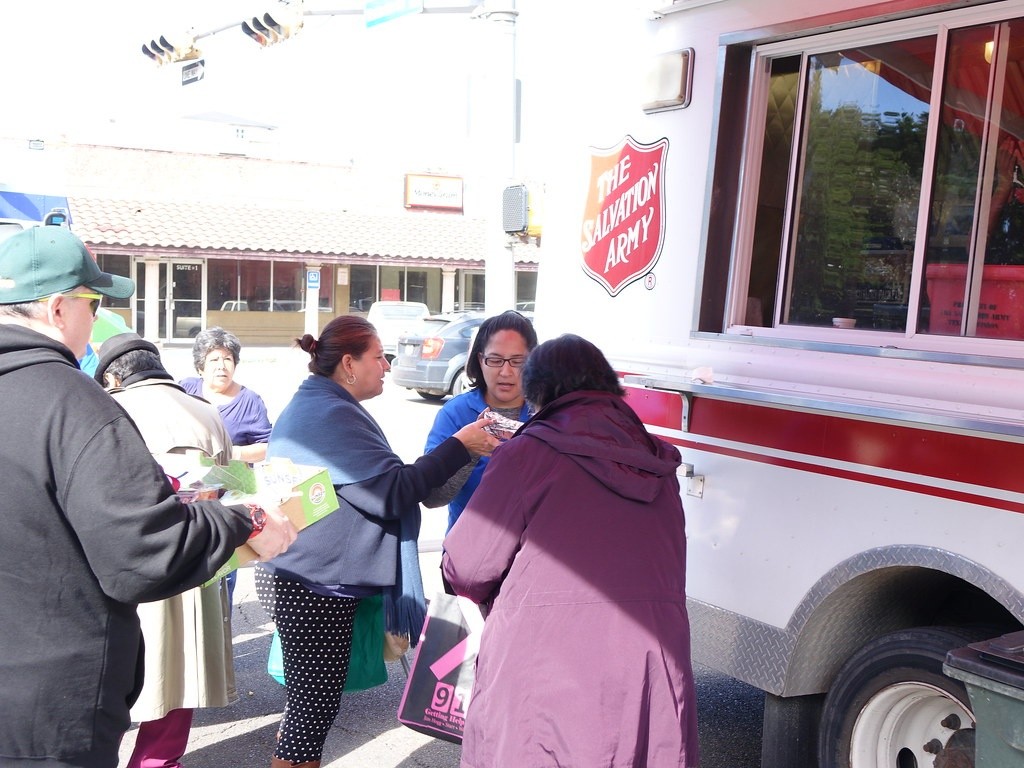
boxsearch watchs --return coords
[243,503,267,540]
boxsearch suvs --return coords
[390,310,533,404]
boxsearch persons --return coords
[258,315,504,768]
[0,216,298,768]
[440,335,701,768]
[420,310,540,537]
[832,113,1024,330]
[176,327,274,619]
[94,330,239,768]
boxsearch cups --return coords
[831,318,856,329]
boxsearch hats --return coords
[0,225,136,305]
[94,332,160,385]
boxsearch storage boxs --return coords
[160,461,342,589]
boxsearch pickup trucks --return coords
[176,301,285,338]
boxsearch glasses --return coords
[482,354,526,367]
[526,405,535,419]
[39,294,103,316]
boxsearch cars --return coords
[366,301,430,362]
[137,310,145,338]
[268,300,320,311]
[518,301,534,310]
[298,305,362,312]
[455,302,485,309]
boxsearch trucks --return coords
[530,0,1024,768]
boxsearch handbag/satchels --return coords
[396,592,489,744]
[267,594,389,692]
